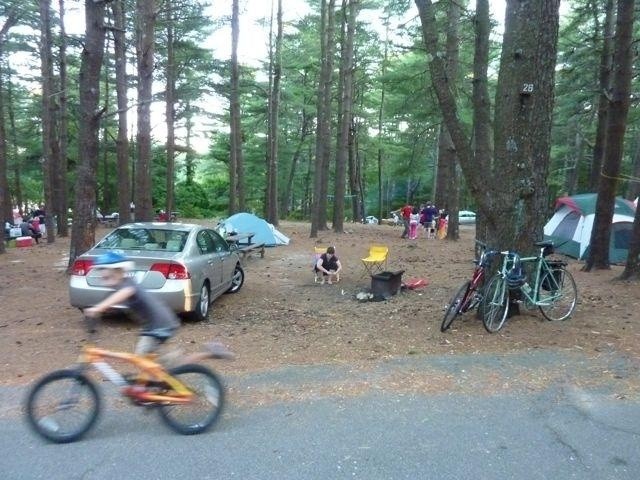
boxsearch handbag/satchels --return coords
[506,259,527,289]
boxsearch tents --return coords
[215,212,289,246]
[543,193,640,266]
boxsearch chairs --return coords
[119,237,138,249]
[314,243,338,280]
[166,238,185,253]
[359,244,390,280]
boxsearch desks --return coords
[4,225,39,243]
[97,216,120,228]
[225,231,255,259]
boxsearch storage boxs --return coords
[17,235,34,247]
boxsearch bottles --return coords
[523,283,531,294]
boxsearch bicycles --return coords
[18,316,228,445]
[438,238,502,334]
[479,238,578,334]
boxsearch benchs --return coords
[4,233,41,246]
[238,241,265,262]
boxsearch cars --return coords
[444,209,477,224]
[154,208,176,221]
[64,220,248,326]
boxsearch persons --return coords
[19,203,47,244]
[216,218,227,236]
[390,201,448,239]
[314,247,342,285]
[83,252,235,371]
